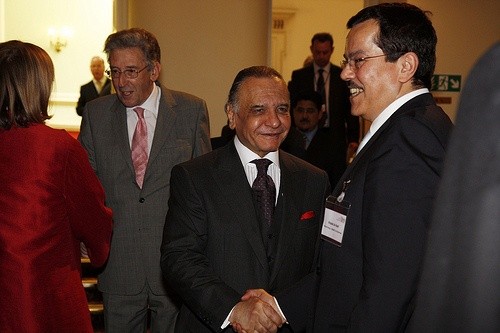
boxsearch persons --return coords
[230,3,456,333]
[77,27,213,332]
[0,40,111,333]
[406,40,500,333]
[76,56,112,117]
[221,33,360,191]
[161,67,328,333]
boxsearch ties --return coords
[130,108,148,190]
[299,134,308,151]
[251,159,276,247]
[315,69,328,113]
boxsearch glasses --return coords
[105,63,151,79]
[338,52,405,69]
